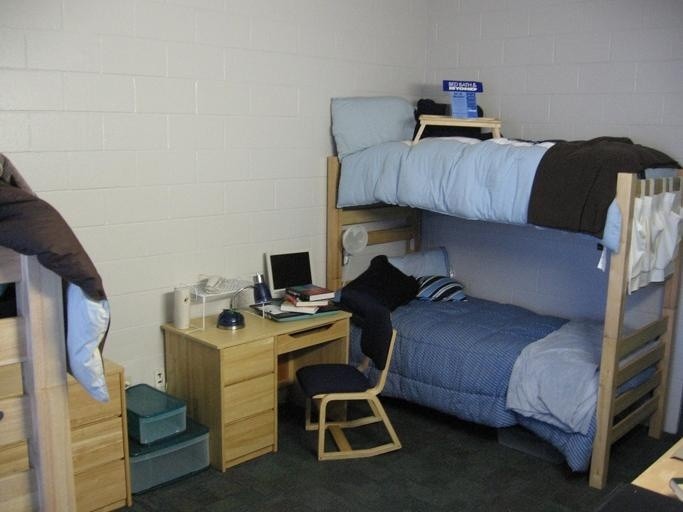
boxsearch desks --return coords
[158,302,351,473]
[410,114,506,148]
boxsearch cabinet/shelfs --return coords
[64,355,133,512]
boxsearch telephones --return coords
[204,274,223,292]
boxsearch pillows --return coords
[331,93,418,160]
[383,244,467,305]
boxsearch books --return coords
[279,300,319,314]
[285,293,328,307]
[285,283,335,301]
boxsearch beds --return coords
[323,150,681,491]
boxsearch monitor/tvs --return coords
[264,247,313,299]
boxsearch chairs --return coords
[294,323,403,462]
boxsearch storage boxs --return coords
[125,384,213,496]
